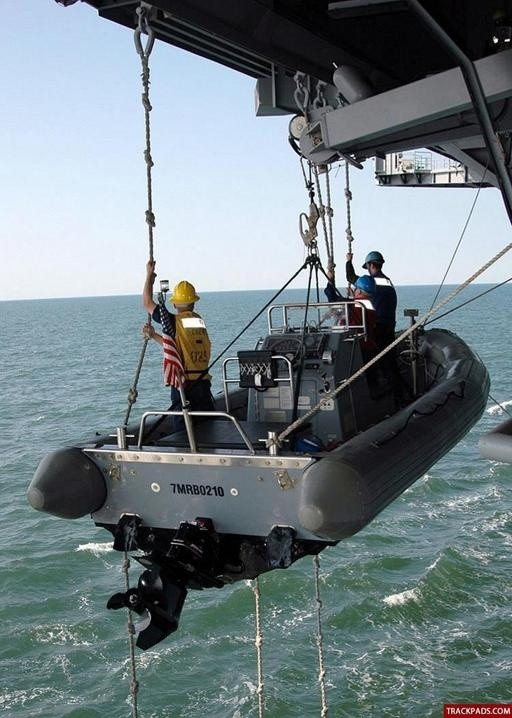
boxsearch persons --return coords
[141,261,215,431]
[346,251,397,344]
[324,266,376,358]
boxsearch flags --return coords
[157,292,187,391]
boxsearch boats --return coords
[26,299,492,653]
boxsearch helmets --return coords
[169,281,200,305]
[354,275,376,294]
[361,251,384,269]
